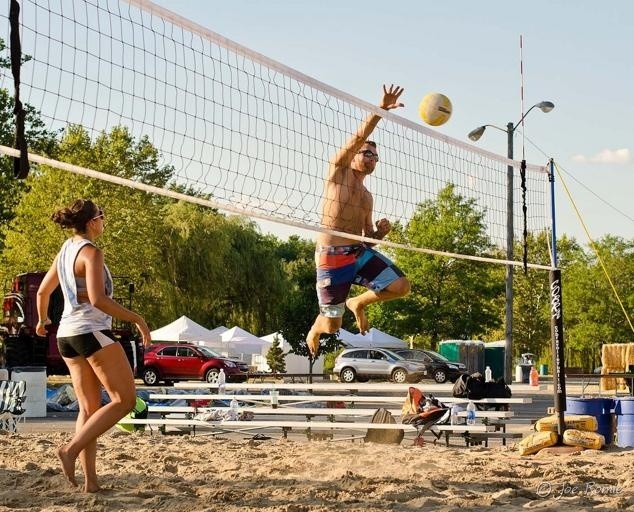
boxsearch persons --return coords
[31,195,154,494]
[303,81,411,360]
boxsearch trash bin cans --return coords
[540,364,548,375]
[11,366,47,418]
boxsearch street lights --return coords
[467,96,564,386]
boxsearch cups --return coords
[269,391,279,409]
[56,385,78,407]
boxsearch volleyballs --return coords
[419,93,452,127]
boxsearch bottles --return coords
[451,406,458,425]
[529,366,538,387]
[485,366,492,382]
[230,397,239,420]
[466,400,476,425]
[218,368,226,395]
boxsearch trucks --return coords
[0,270,140,377]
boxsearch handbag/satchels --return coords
[113,395,147,432]
[453,371,513,399]
[402,386,452,425]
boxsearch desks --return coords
[565,373,634,398]
[243,372,327,383]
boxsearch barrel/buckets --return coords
[564,396,614,444]
[613,398,634,449]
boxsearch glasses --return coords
[357,149,380,163]
[92,211,104,220]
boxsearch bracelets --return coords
[37,318,48,324]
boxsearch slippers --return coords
[243,433,271,440]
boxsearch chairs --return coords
[0,380,27,434]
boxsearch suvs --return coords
[394,347,470,383]
[136,342,252,386]
[332,346,428,385]
